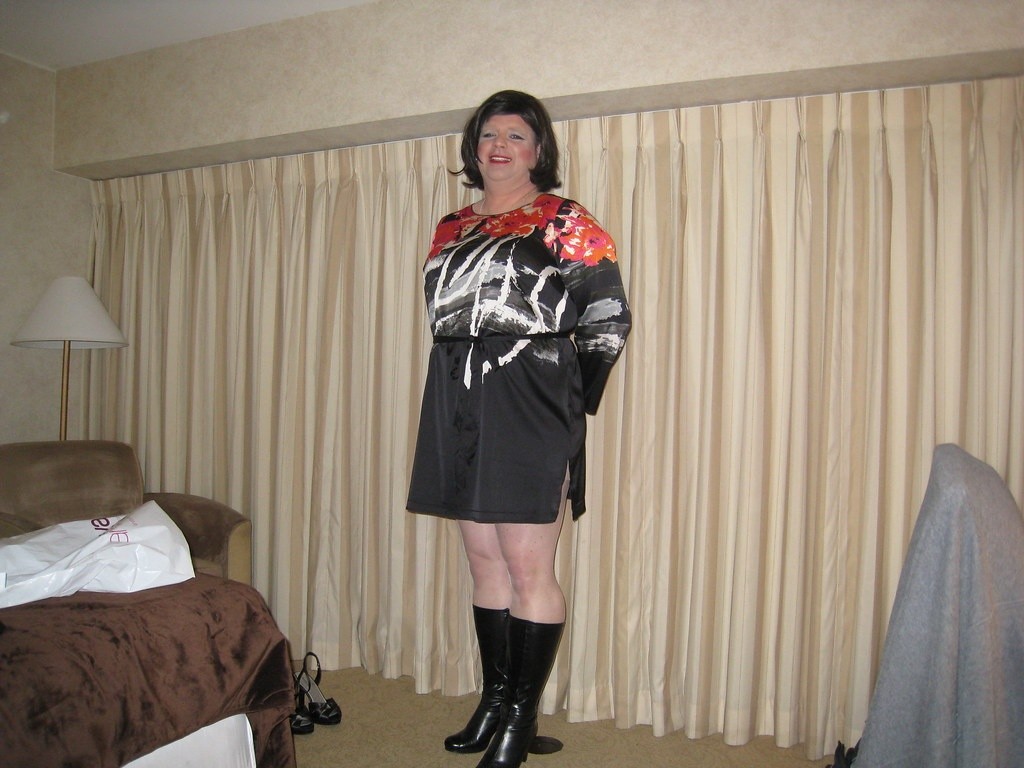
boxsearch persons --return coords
[406,91,633,768]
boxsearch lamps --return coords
[10,276,131,443]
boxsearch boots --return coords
[475,612,567,768]
[443,602,511,754]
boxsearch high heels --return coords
[293,652,343,725]
[289,672,314,735]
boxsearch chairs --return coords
[0,440,300,768]
